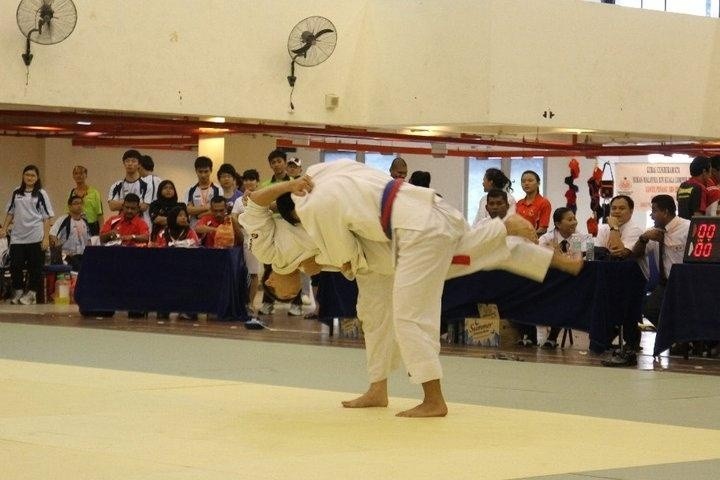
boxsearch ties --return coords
[659,240,664,278]
[560,240,567,253]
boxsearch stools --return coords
[41,265,73,305]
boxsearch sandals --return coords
[304,312,320,318]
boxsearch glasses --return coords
[72,203,81,205]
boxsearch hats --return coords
[287,156,301,167]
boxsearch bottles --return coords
[64,275,73,303]
[55,275,70,307]
[586,233,595,261]
[571,234,582,260]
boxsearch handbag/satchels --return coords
[599,178,614,198]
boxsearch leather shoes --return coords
[602,352,637,366]
[589,346,618,355]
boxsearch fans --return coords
[286,15,337,87]
[15,0,77,68]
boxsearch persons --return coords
[2,148,431,332]
[277,158,583,417]
[475,156,720,364]
[239,176,540,408]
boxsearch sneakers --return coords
[518,338,537,346]
[542,340,557,349]
[246,304,255,316]
[288,304,303,316]
[19,290,36,305]
[11,290,22,304]
[180,313,191,319]
[259,302,275,314]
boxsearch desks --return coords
[652,264,719,360]
[315,261,636,362]
[73,247,244,322]
